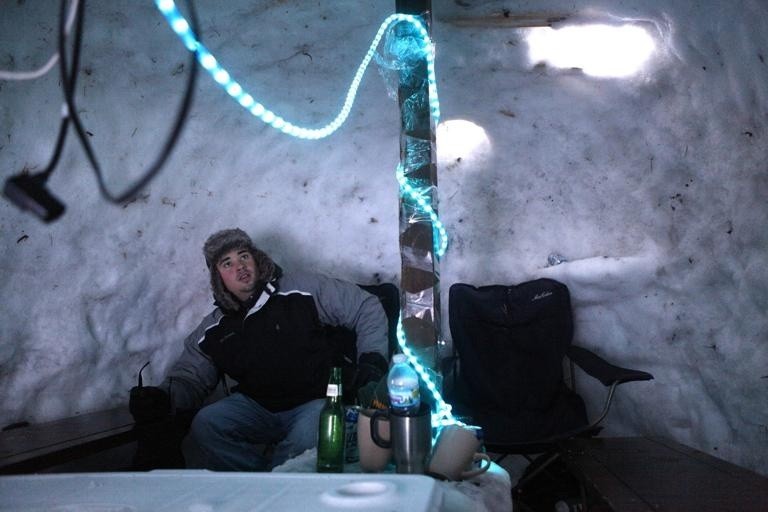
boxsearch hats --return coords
[202,227,282,311]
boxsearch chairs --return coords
[323,283,400,366]
[436,277,654,467]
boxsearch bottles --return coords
[389,354,421,409]
[317,366,347,472]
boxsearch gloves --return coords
[129,386,170,428]
[350,351,389,405]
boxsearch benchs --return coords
[555,432,768,512]
[0,387,230,474]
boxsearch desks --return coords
[272,442,515,512]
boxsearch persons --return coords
[126,225,391,472]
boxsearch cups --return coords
[358,401,490,481]
